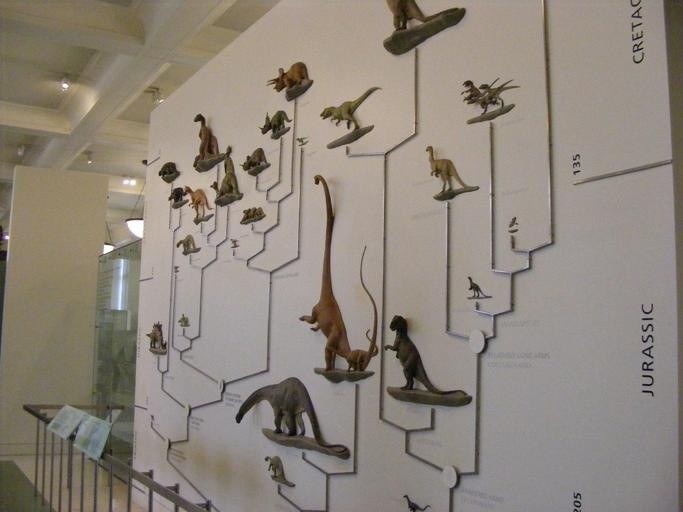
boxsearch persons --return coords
[93,345,122,416]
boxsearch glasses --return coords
[144,84,165,107]
[100,219,115,254]
[124,174,146,239]
[83,151,95,164]
[59,73,71,89]
